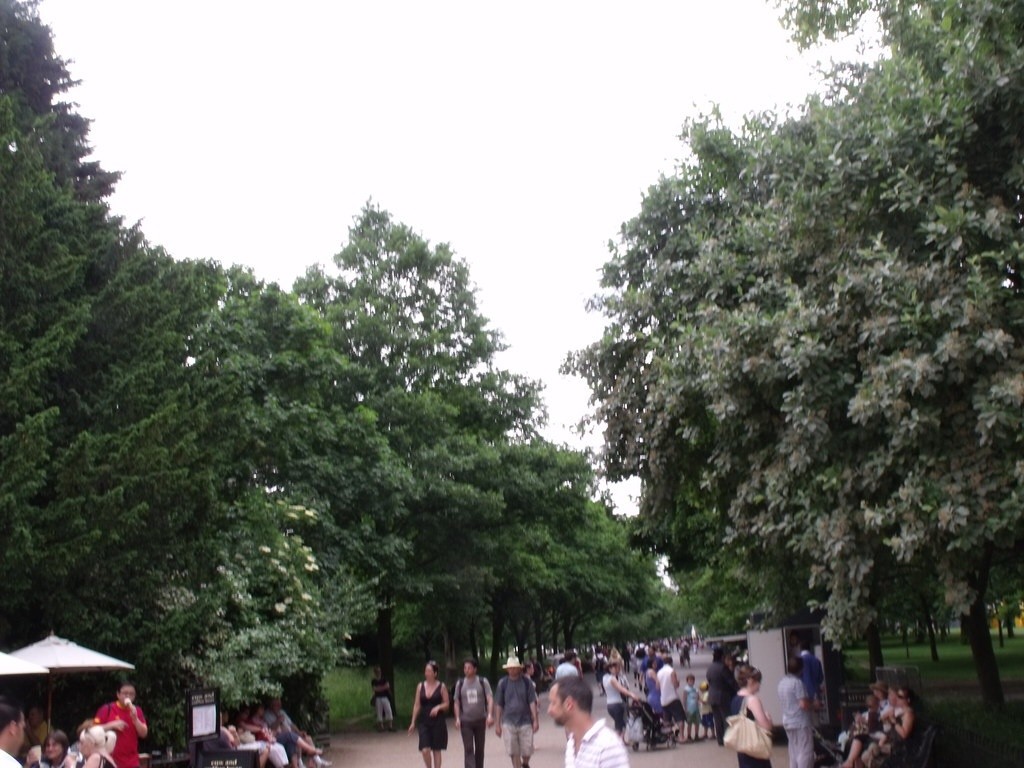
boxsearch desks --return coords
[152,753,191,768]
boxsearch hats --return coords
[501,655,521,669]
[869,681,888,693]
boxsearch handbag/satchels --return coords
[624,712,644,744]
[723,695,772,759]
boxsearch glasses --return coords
[429,661,437,668]
[896,693,905,700]
[121,691,136,699]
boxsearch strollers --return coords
[625,696,676,751]
[810,725,846,768]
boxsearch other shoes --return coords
[379,728,387,731]
[317,760,333,768]
[678,734,717,746]
[389,727,396,732]
[839,760,856,768]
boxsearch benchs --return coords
[883,721,945,768]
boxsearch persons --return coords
[843,679,915,768]
[0,682,147,768]
[453,658,494,768]
[547,676,631,768]
[494,656,543,767]
[553,651,578,740]
[202,696,333,768]
[682,674,704,743]
[592,647,608,696]
[621,634,720,674]
[796,641,824,767]
[408,661,450,768]
[371,666,398,733]
[730,665,774,768]
[778,657,823,768]
[603,649,716,746]
[706,648,737,747]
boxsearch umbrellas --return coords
[7,629,135,736]
[0,651,50,675]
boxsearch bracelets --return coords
[892,721,899,727]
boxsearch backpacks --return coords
[595,653,605,673]
